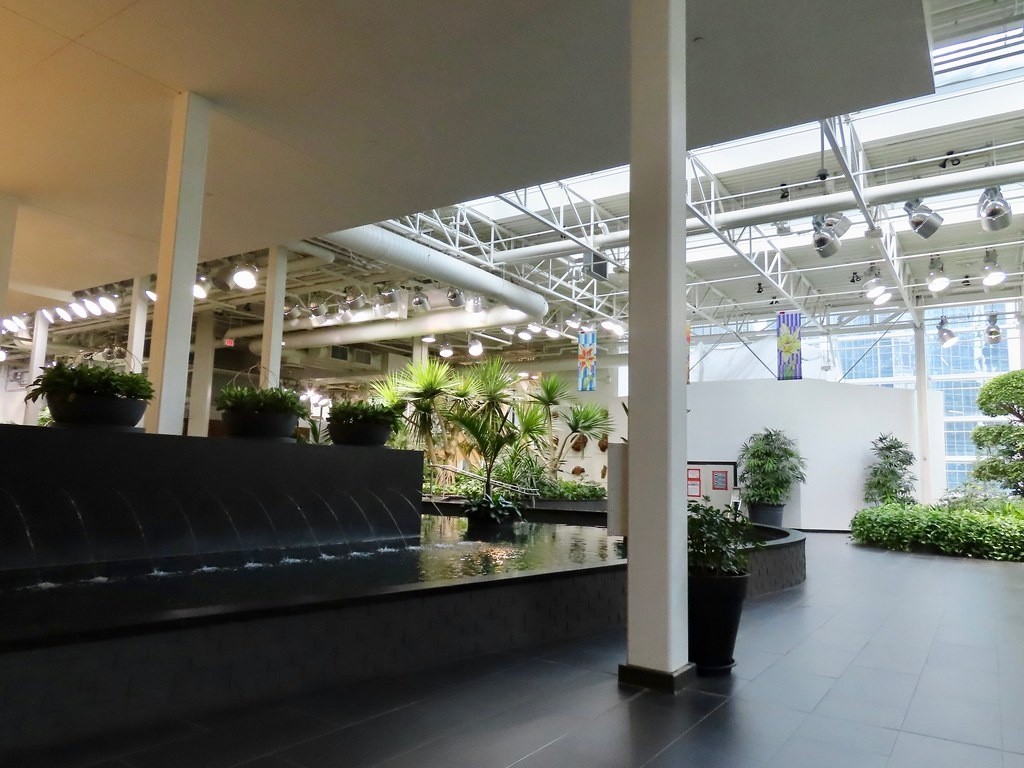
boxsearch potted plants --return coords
[325,401,400,449]
[688,493,767,677]
[24,358,158,432]
[735,425,809,528]
[442,353,527,539]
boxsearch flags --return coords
[774,311,803,380]
[577,330,598,391]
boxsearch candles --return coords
[214,384,311,443]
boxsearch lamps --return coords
[860,264,886,299]
[980,248,1007,287]
[212,266,238,291]
[233,266,258,290]
[871,290,891,306]
[985,314,1002,344]
[2,288,126,332]
[936,314,959,348]
[976,186,1012,233]
[193,280,212,298]
[902,196,944,240]
[821,210,853,239]
[812,214,841,258]
[145,287,157,303]
[926,252,951,292]
[282,282,625,357]
[0,351,7,363]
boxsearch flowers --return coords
[778,323,802,379]
[578,343,596,391]
